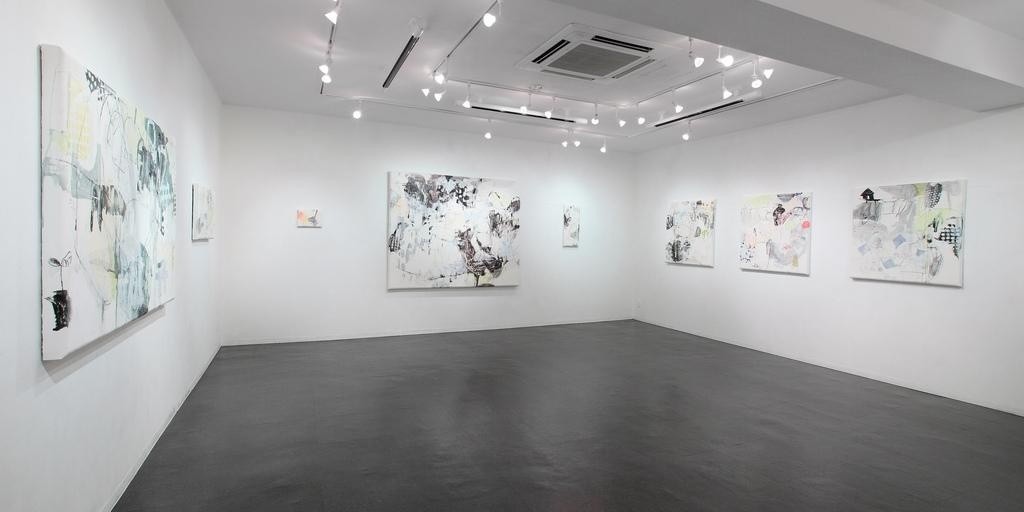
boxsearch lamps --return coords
[347,105,613,156]
[313,0,345,89]
[419,82,604,126]
[670,122,696,143]
[613,57,778,129]
[685,33,736,70]
[432,2,502,84]
